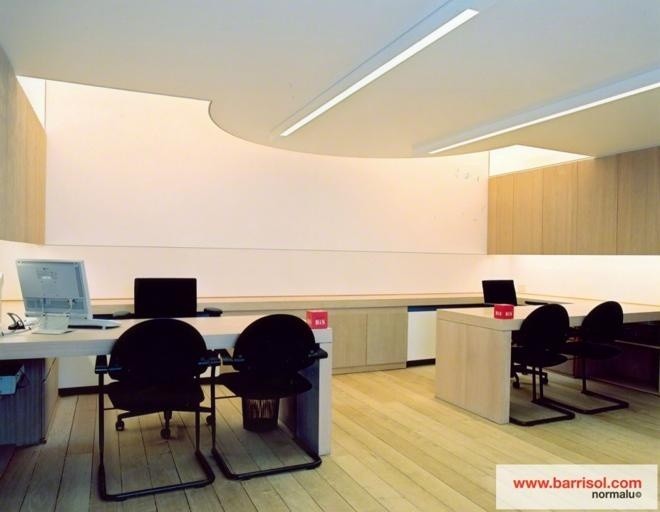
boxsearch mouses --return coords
[112,309,129,317]
[204,306,223,315]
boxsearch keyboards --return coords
[70,318,124,332]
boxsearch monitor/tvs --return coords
[134,278,198,316]
[482,279,517,306]
[16,258,93,321]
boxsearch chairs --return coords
[482,278,548,389]
[540,300,629,416]
[508,303,575,427]
[94,318,220,502]
[108,277,223,440]
[212,314,322,481]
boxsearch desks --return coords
[0,313,332,458]
[433,300,660,424]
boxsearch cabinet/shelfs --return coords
[0,358,59,448]
[330,307,408,375]
[618,145,660,254]
[541,154,620,254]
[486,167,543,255]
[1,47,47,246]
